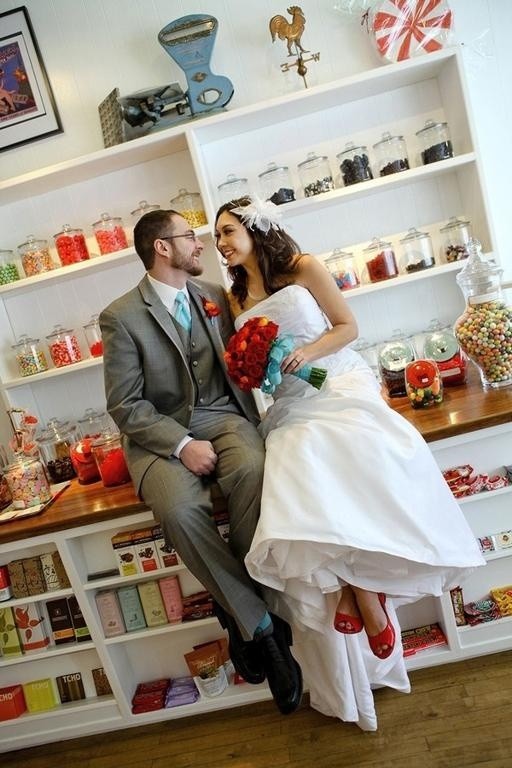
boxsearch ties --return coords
[171,290,195,333]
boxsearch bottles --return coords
[419,315,471,389]
[370,130,410,177]
[34,406,132,488]
[170,188,206,229]
[91,213,129,256]
[374,331,418,405]
[1,451,53,506]
[256,159,296,206]
[0,248,21,287]
[323,249,360,291]
[45,321,83,366]
[17,234,54,276]
[412,121,456,165]
[10,333,49,375]
[53,224,91,266]
[397,226,436,272]
[294,151,333,194]
[439,214,473,260]
[217,172,256,205]
[402,357,444,407]
[450,235,512,391]
[362,237,400,283]
[82,313,106,359]
[332,141,374,183]
[128,199,162,227]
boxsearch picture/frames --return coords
[0,5,64,153]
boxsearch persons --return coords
[211,192,486,659]
[101,207,305,716]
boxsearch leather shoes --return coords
[333,610,361,638]
[206,598,267,688]
[260,610,305,719]
[361,588,396,662]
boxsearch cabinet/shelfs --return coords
[0,512,275,753]
[396,424,511,671]
[0,46,510,469]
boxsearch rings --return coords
[293,358,300,364]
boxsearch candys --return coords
[0,433,131,510]
[15,335,104,377]
[0,225,128,286]
[178,208,208,229]
[264,140,453,205]
[332,245,470,291]
[380,299,512,407]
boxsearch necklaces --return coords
[246,284,270,303]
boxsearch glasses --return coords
[157,229,200,243]
